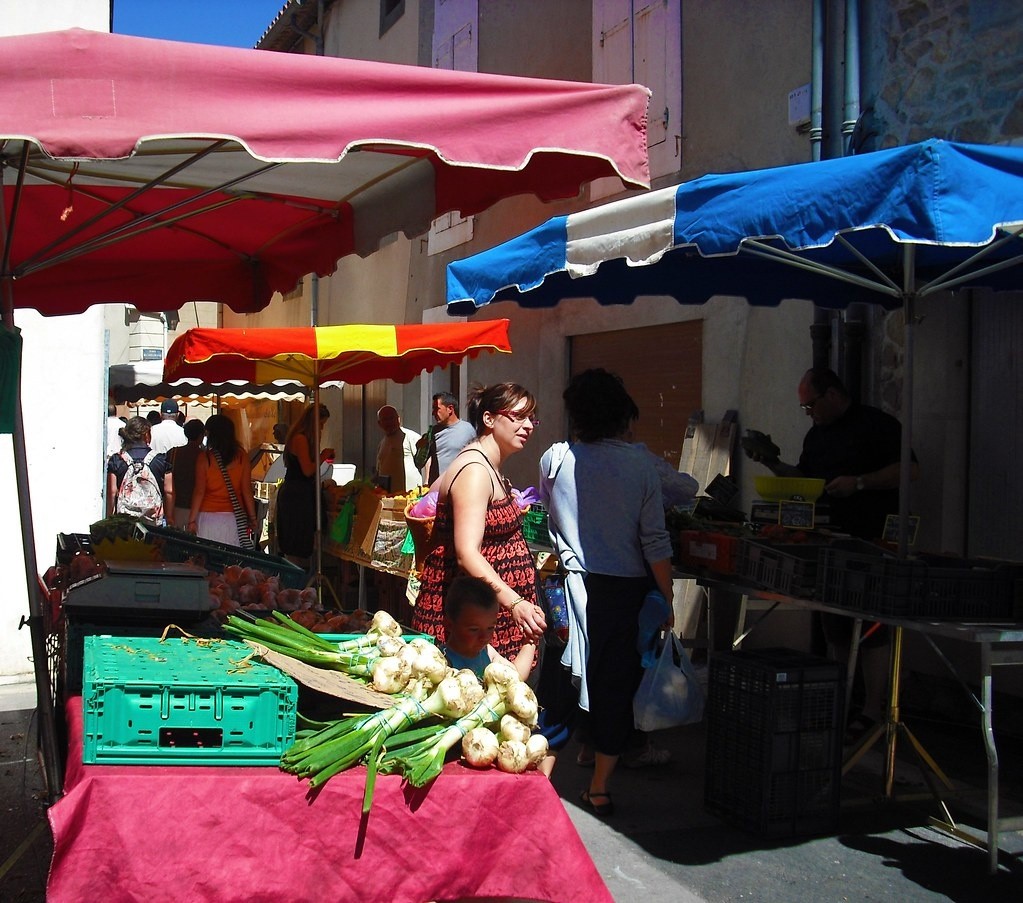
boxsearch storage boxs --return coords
[82,610,435,766]
[681,531,1023,842]
[522,503,552,546]
[56,522,305,590]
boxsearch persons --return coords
[410,380,546,672]
[188,414,257,549]
[438,576,546,686]
[106,399,205,532]
[274,403,335,569]
[375,405,432,493]
[536,367,676,812]
[739,364,924,746]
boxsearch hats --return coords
[161,398,178,413]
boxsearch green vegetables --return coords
[91,512,138,541]
[664,510,755,543]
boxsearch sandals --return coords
[579,788,613,816]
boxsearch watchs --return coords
[855,474,868,492]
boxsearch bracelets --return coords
[510,597,525,610]
[188,521,196,525]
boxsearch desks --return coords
[696,576,1021,875]
[314,542,424,612]
[46,696,614,903]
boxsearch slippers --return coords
[622,743,671,768]
[577,752,595,766]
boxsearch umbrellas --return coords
[165,320,513,612]
[108,359,346,416]
[444,136,1023,566]
[0,31,652,797]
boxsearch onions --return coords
[371,610,550,772]
[206,566,369,633]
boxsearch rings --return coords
[524,625,529,630]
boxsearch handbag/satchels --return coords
[234,509,255,550]
[534,569,570,649]
[329,492,356,545]
[404,490,531,582]
[632,625,706,732]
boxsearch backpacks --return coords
[116,448,165,528]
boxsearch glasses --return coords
[800,393,824,410]
[495,409,540,427]
